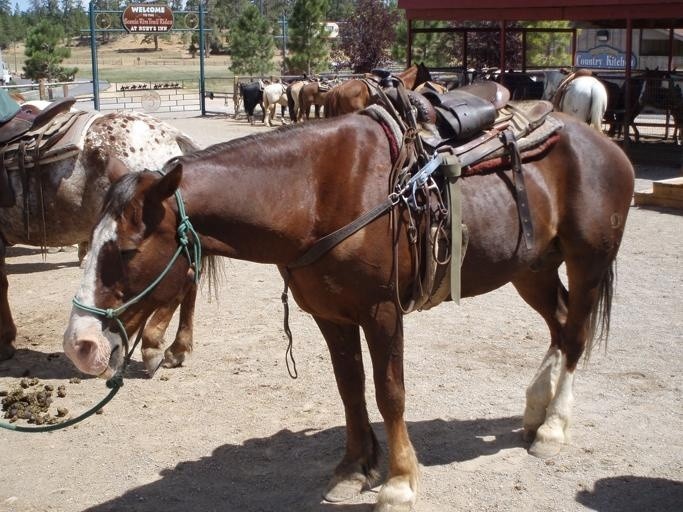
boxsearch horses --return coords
[62,96,635,512]
[0,107,237,381]
[236,60,683,147]
